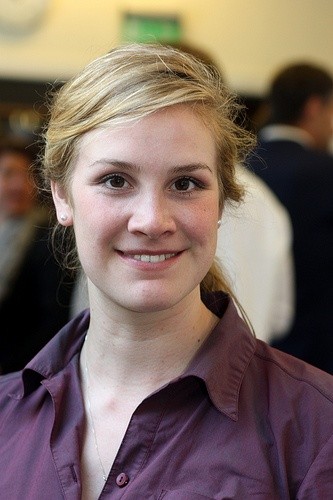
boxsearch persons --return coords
[1,39,333,499]
[73,41,296,349]
[242,58,332,378]
[0,141,77,378]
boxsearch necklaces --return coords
[82,331,108,486]
